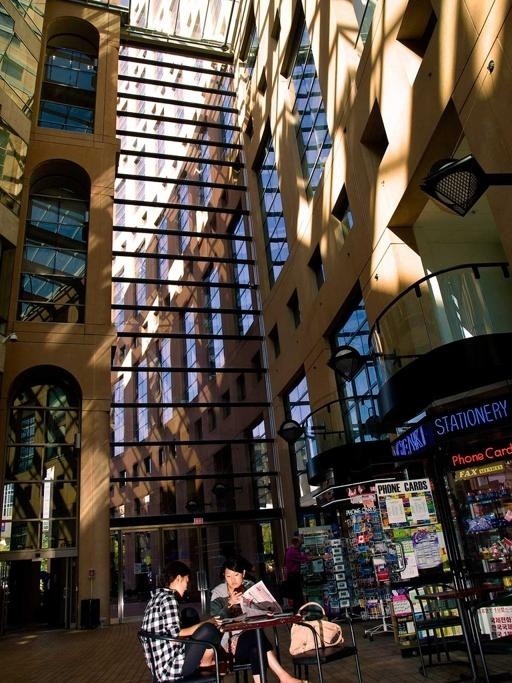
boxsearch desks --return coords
[414,584,505,683]
[220,614,303,683]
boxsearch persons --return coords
[285,531,331,617]
[209,557,308,683]
[141,561,223,683]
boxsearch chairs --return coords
[137,630,219,683]
[285,606,362,683]
[470,593,512,683]
[404,583,470,677]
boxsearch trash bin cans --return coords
[81,599,101,630]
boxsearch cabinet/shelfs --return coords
[454,460,512,609]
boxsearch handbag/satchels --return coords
[289,619,345,658]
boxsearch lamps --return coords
[418,152,512,219]
[2,332,18,344]
